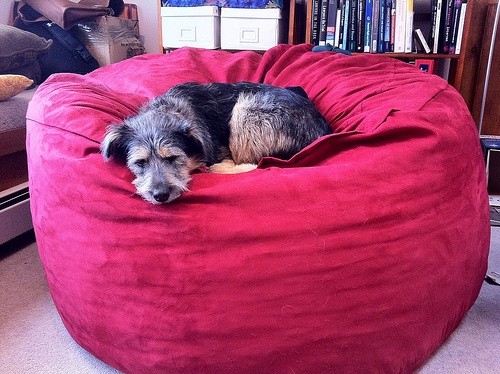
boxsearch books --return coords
[415,29,431,54]
[430,0,467,55]
[310,0,415,54]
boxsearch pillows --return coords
[0,23,54,75]
[0,74,35,101]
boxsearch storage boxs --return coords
[161,6,220,50]
[219,7,283,51]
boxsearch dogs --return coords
[100,81,334,203]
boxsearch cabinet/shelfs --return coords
[288,0,486,128]
